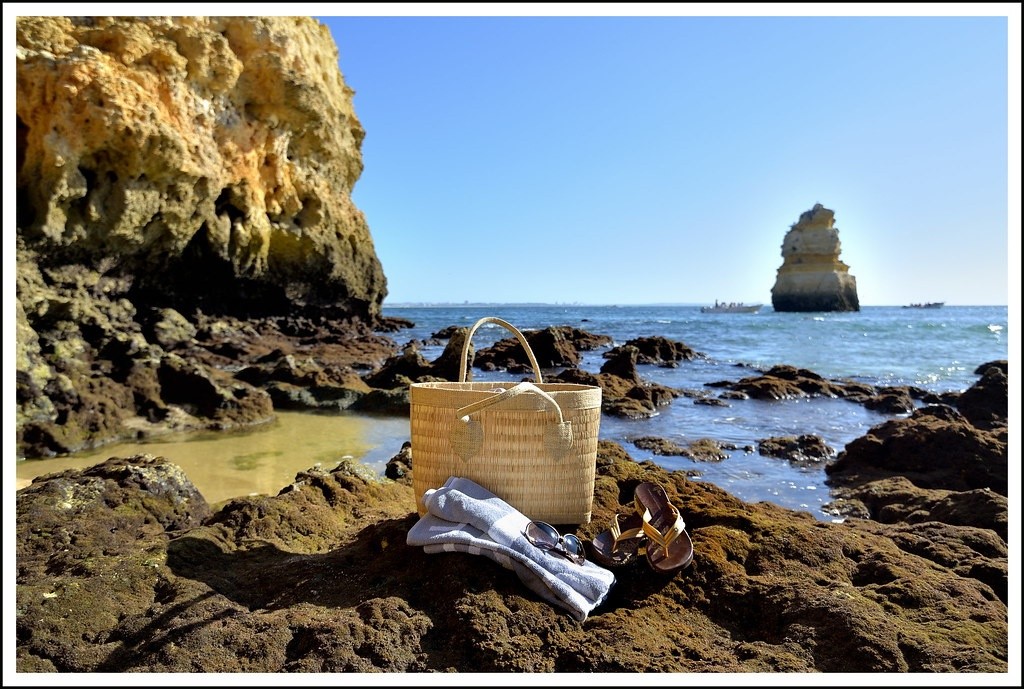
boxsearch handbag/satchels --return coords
[411,316,605,525]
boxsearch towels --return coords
[405,475,617,623]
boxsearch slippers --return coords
[633,483,694,576]
[591,511,644,567]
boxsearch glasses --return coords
[525,521,587,567]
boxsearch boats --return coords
[902,302,945,309]
[699,299,765,313]
